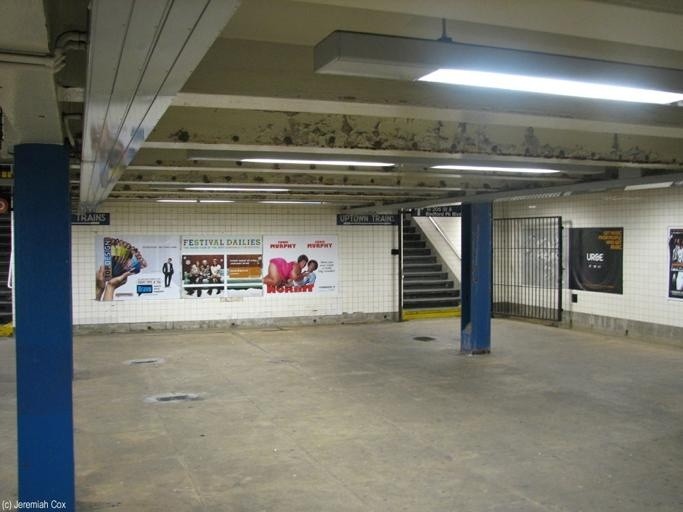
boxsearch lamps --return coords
[312,15,682,106]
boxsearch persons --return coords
[93,240,146,302]
[288,260,318,286]
[161,258,174,287]
[184,258,226,296]
[264,255,309,287]
